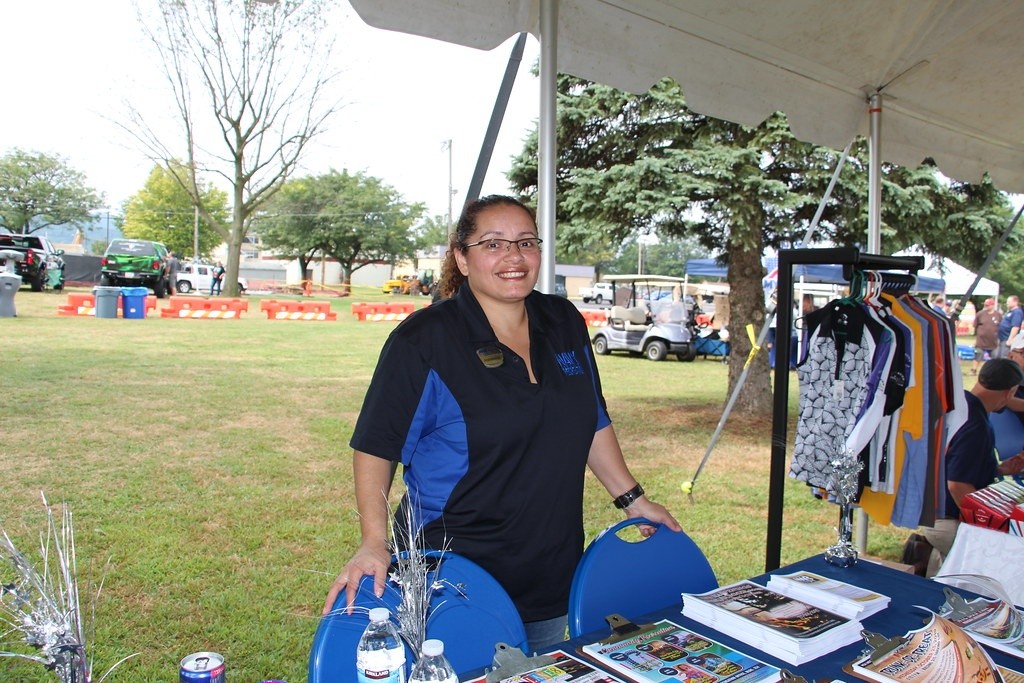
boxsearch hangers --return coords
[795,270,934,342]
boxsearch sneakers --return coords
[901,533,933,578]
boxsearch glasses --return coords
[466,238,543,253]
[1012,350,1024,357]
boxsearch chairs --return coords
[568,517,720,640]
[307,548,529,683]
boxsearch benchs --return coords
[610,305,649,331]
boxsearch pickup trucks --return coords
[579,283,619,304]
[177,265,247,293]
[0,233,66,290]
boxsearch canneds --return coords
[179,651,226,683]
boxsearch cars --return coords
[555,284,567,297]
[100,239,171,298]
[659,294,693,304]
[644,291,671,300]
[383,273,409,293]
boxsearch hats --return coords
[981,298,995,305]
[1011,335,1024,350]
[978,358,1024,390]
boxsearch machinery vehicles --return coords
[400,267,440,297]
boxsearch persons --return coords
[931,296,1023,577]
[320,195,685,657]
[801,294,818,314]
[163,251,178,296]
[209,261,225,295]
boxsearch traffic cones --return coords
[117,296,123,318]
[303,279,314,298]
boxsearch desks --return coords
[454,552,1024,683]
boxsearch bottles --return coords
[407,639,459,683]
[356,607,406,683]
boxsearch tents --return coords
[683,255,789,304]
[889,241,1000,319]
[789,262,945,365]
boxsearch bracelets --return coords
[612,484,645,509]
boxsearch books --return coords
[465,568,892,683]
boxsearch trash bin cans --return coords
[0,272,22,316]
[92,286,121,318]
[121,287,147,319]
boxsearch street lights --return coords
[441,139,457,247]
